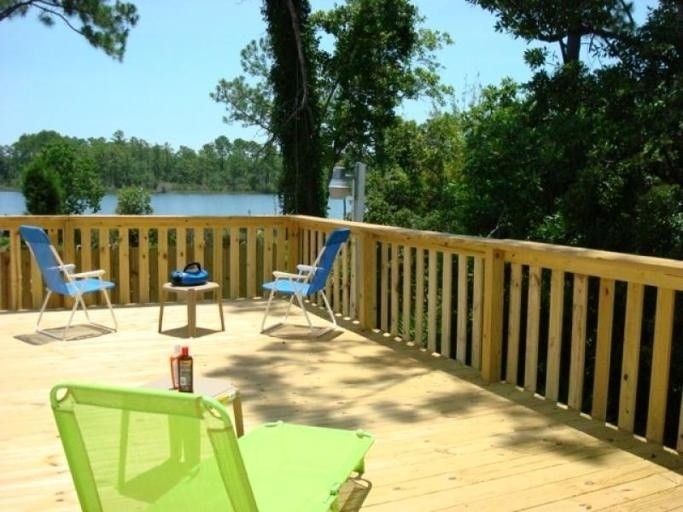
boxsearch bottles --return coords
[170,344,193,392]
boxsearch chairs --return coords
[49,385,374,512]
[19,225,119,339]
[261,229,351,338]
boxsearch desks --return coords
[118,377,245,487]
[158,283,225,339]
